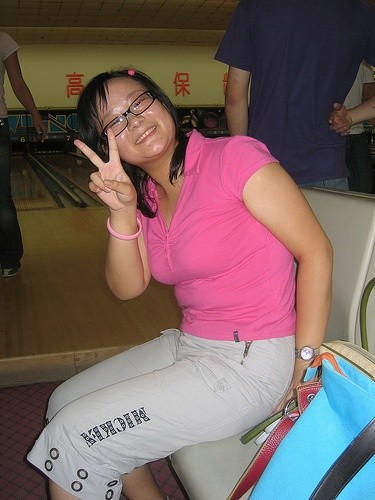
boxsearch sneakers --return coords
[0,261,21,278]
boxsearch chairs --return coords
[165,187,375,500]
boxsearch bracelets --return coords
[106,217,142,240]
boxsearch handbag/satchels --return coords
[227,339,375,500]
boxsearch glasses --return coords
[100,90,156,145]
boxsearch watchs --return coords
[295,346,320,361]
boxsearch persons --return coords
[0,32,47,277]
[212,0,375,191]
[25,64,335,500]
